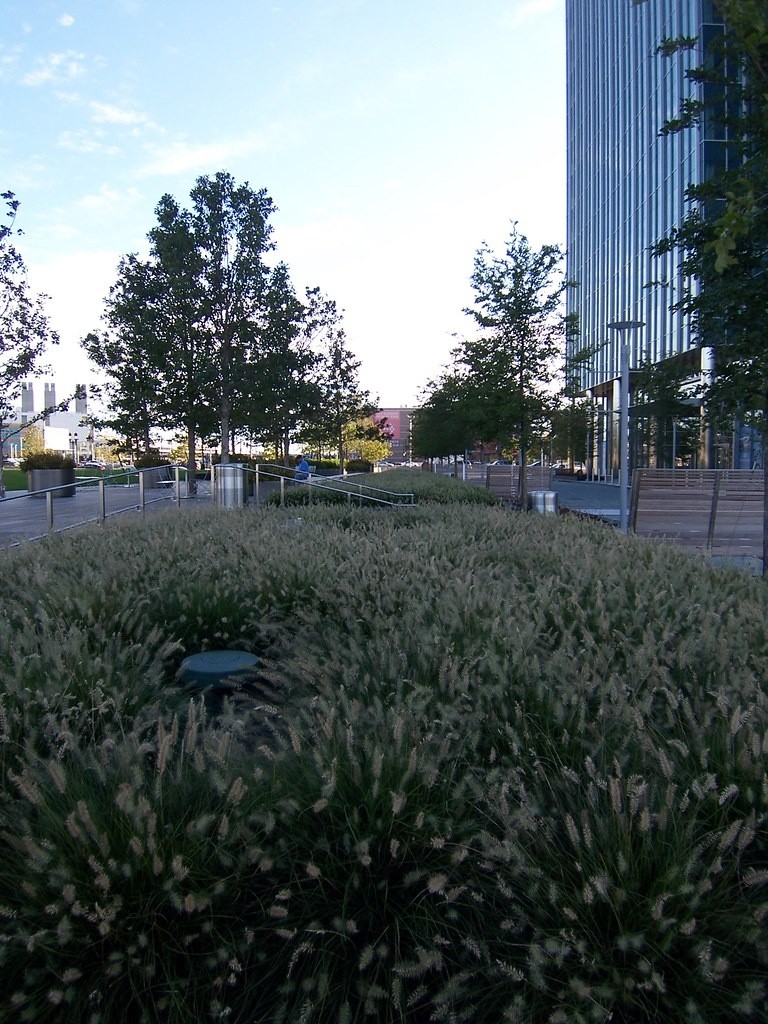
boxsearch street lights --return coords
[69,433,79,459]
[406,414,416,468]
[603,320,649,537]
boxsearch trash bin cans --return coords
[215,463,249,509]
[528,490,560,515]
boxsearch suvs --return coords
[527,461,587,474]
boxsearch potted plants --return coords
[134,457,173,489]
[19,452,76,497]
[345,459,374,474]
[212,453,257,497]
[310,460,340,476]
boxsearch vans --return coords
[492,460,518,465]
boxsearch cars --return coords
[379,460,401,467]
[177,461,201,470]
[4,456,26,467]
[76,461,122,471]
[450,460,482,468]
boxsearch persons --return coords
[294,454,309,487]
